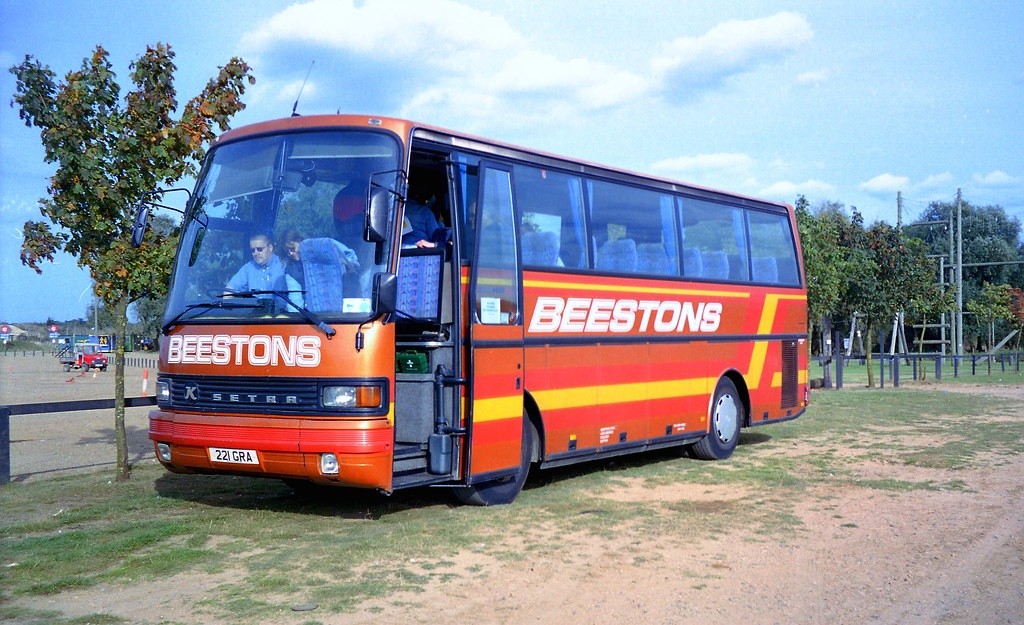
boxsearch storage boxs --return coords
[400,352,429,375]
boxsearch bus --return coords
[130,112,813,508]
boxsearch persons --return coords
[223,234,295,313]
[281,227,360,313]
[402,177,441,247]
[334,159,376,298]
[140,336,153,350]
[429,171,565,266]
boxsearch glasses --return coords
[286,242,299,253]
[251,244,269,252]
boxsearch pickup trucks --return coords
[60,343,108,372]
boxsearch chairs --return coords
[396,246,446,324]
[518,230,779,286]
[297,240,343,312]
